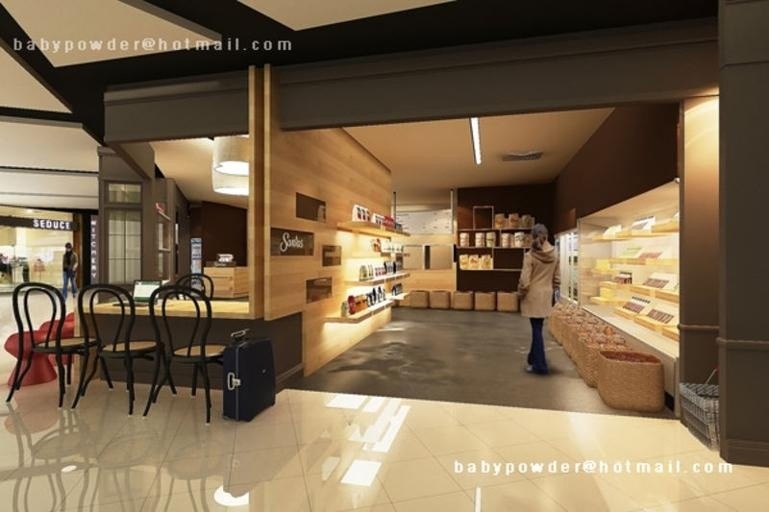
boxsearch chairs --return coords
[7,281,226,425]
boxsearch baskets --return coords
[547,303,663,412]
[409,289,522,312]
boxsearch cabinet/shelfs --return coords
[324,221,411,323]
[457,205,535,271]
[591,231,680,343]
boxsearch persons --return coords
[61,242,79,300]
[518,222,561,374]
